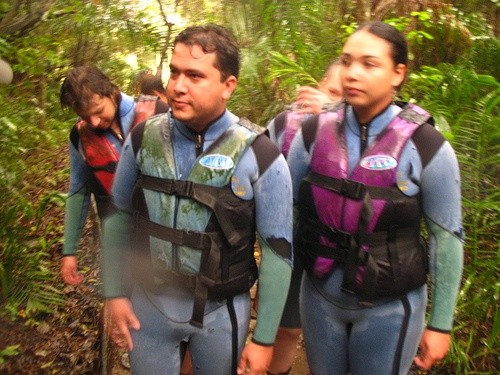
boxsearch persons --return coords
[100,21,294,375]
[263,57,344,375]
[58,62,169,286]
[285,22,464,375]
[134,71,169,105]
